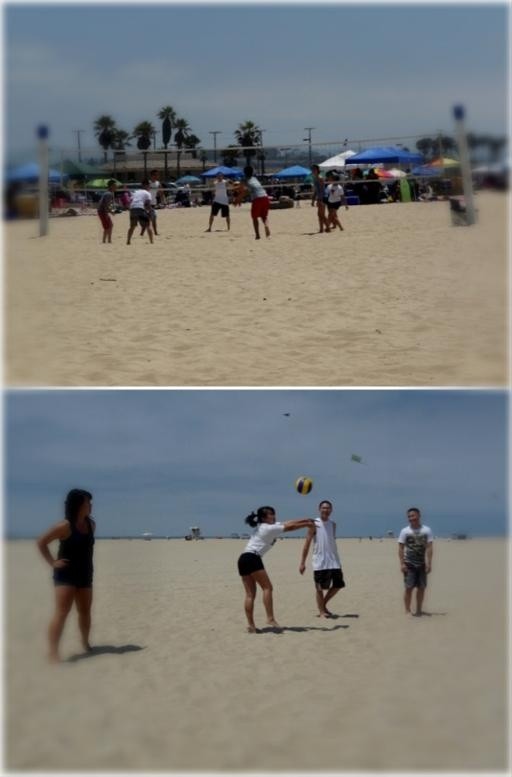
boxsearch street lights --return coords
[208,129,222,163]
[342,138,349,149]
[279,147,291,169]
[304,127,314,168]
[71,129,88,162]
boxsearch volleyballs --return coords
[296,476,312,494]
[232,181,240,188]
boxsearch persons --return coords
[298,500,345,617]
[395,510,433,616]
[35,489,96,665]
[237,507,321,634]
